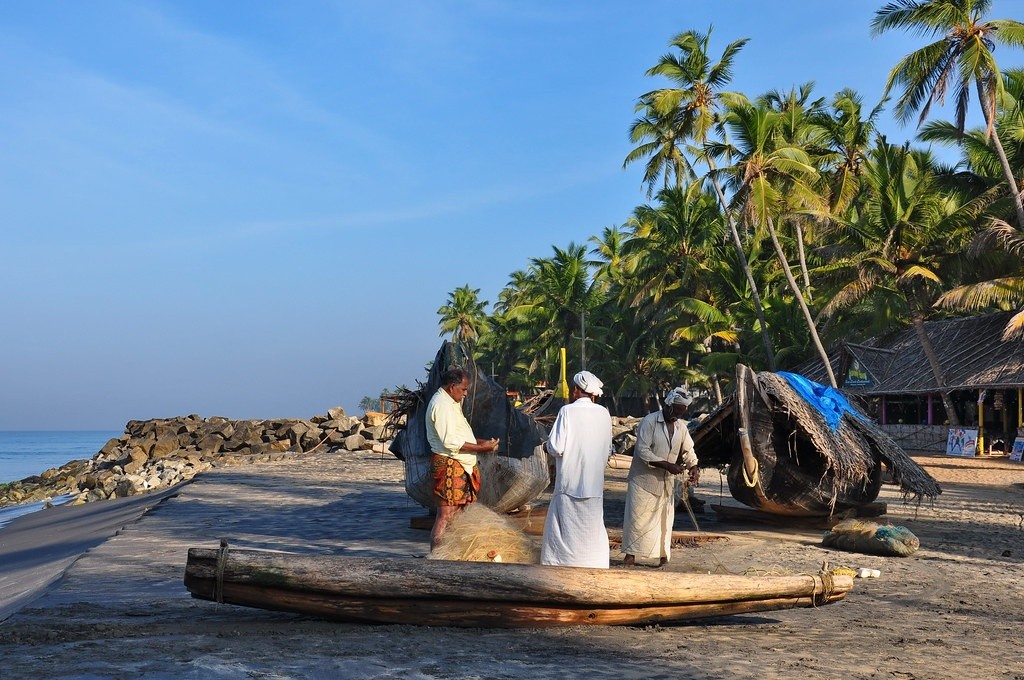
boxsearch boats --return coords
[726,363,882,518]
[388,339,571,516]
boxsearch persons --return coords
[426,370,500,551]
[621,386,699,570]
[539,371,612,569]
